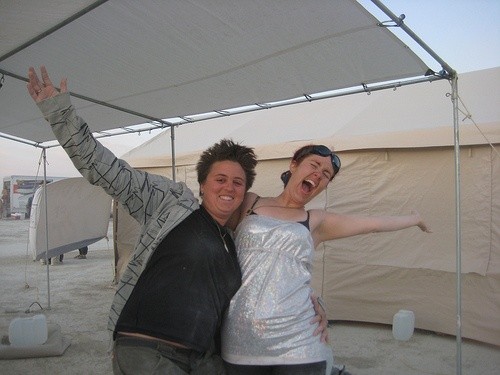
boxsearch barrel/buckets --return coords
[9,314,48,344]
[393,310,415,340]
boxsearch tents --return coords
[0,0,500,375]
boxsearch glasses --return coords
[297,144,342,169]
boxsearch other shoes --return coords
[74,253,87,260]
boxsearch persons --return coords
[219,145,434,375]
[25,64,329,375]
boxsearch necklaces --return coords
[212,218,230,254]
[244,195,306,217]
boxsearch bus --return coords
[3,175,65,221]
